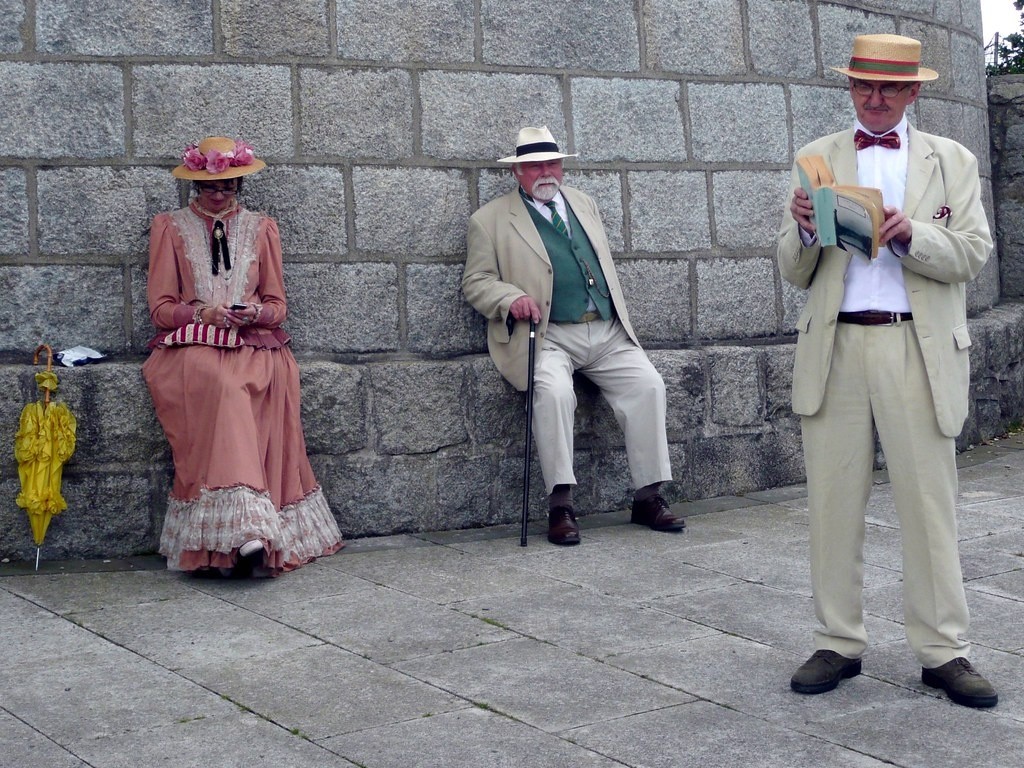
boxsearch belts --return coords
[837,310,913,326]
[548,311,600,324]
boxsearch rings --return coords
[242,315,248,321]
[223,317,230,327]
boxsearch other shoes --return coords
[238,538,268,563]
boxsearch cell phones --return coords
[230,304,247,310]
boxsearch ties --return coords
[544,201,568,237]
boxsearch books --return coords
[796,154,887,259]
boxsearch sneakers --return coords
[922,657,998,708]
[791,650,862,693]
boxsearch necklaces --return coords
[200,204,239,280]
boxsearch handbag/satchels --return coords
[164,298,244,348]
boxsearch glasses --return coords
[198,182,239,195]
[851,79,910,98]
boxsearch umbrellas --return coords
[13,344,77,569]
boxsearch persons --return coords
[462,126,685,545]
[142,136,345,580]
[778,34,998,708]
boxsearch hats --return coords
[172,137,266,180]
[832,34,939,81]
[497,125,578,162]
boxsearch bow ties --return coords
[853,129,900,150]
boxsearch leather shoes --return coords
[631,493,685,530]
[549,505,580,544]
